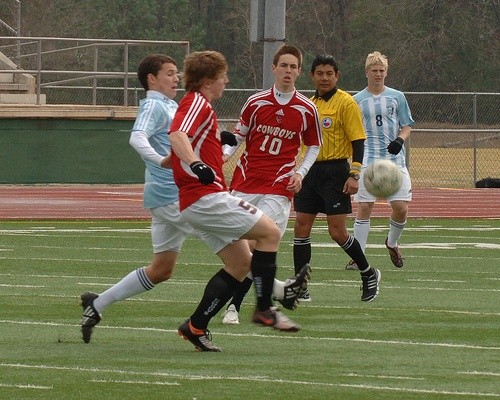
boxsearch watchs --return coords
[350,173,360,180]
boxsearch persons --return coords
[79,54,313,344]
[288,53,381,302]
[345,51,414,270]
[168,49,302,353]
[222,45,322,325]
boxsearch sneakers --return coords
[272,263,313,311]
[385,237,405,268]
[189,161,215,185]
[220,304,240,325]
[79,290,103,344]
[178,317,221,351]
[220,130,237,147]
[345,258,358,270]
[252,303,301,332]
[359,268,381,302]
[386,137,405,155]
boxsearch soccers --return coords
[363,159,403,197]
